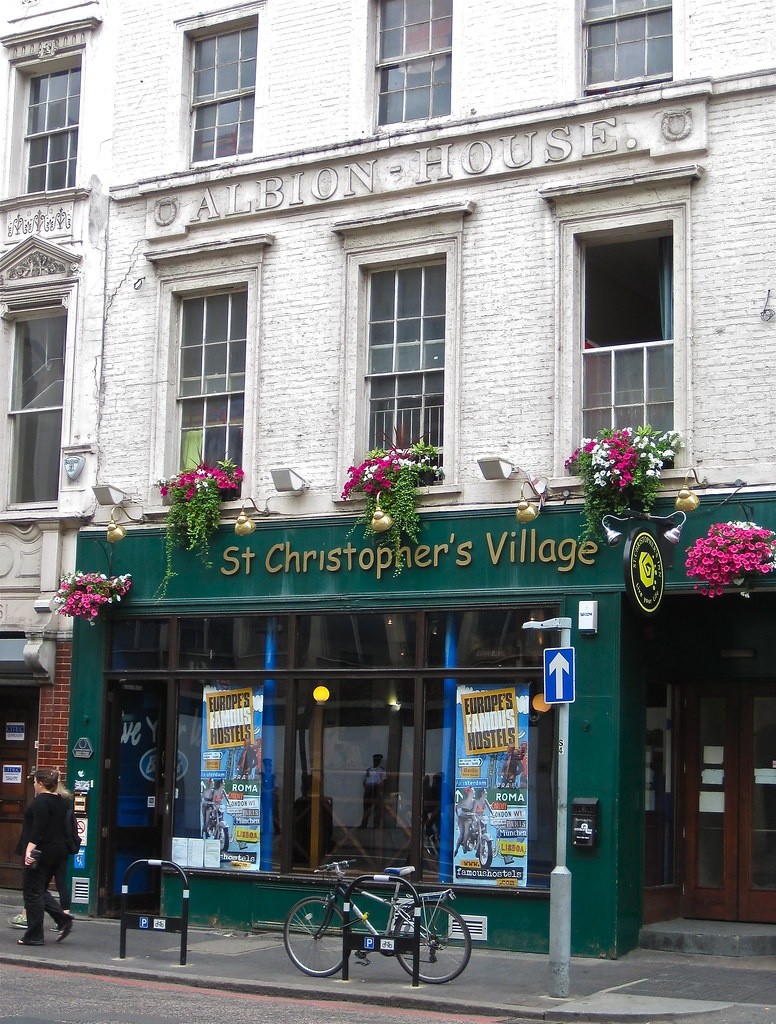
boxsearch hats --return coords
[373,754,383,759]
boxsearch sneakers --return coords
[7,914,28,928]
[49,922,59,931]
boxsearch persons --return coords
[423,773,443,843]
[8,769,83,945]
[497,740,528,790]
[454,786,495,858]
[357,754,386,830]
[202,777,231,836]
[234,736,261,780]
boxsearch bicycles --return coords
[282,859,475,984]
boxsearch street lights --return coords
[519,617,573,1003]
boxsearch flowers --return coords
[152,445,244,605]
[340,423,446,578]
[562,423,684,554]
[686,519,776,598]
[52,570,132,627]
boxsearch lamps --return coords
[107,505,143,544]
[312,683,330,706]
[677,468,709,510]
[516,482,549,523]
[641,511,687,544]
[92,485,131,506]
[478,457,519,479]
[270,467,310,494]
[601,515,644,548]
[371,492,395,534]
[234,497,270,537]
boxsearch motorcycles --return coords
[202,802,230,851]
[458,812,496,868]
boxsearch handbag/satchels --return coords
[65,799,82,855]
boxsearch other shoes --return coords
[16,938,45,945]
[372,826,379,829]
[357,825,367,829]
[56,921,74,943]
